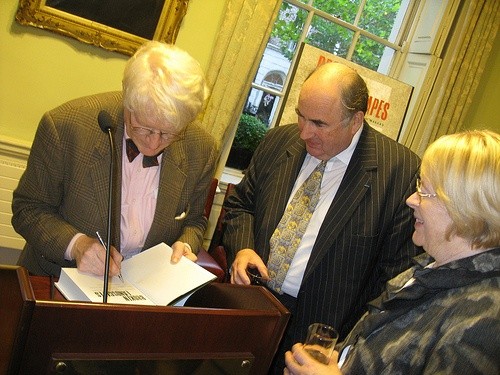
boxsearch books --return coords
[54,242,218,310]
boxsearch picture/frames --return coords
[14,1,189,60]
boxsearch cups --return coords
[246,267,276,291]
[304,323,339,365]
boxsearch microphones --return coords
[97,109,117,303]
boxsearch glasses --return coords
[128,111,184,143]
[416,178,440,198]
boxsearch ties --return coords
[263,161,328,293]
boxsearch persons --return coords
[11,41,218,278]
[221,63,422,375]
[284,129,500,375]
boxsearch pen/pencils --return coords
[96,230,125,280]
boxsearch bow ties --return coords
[125,138,165,168]
